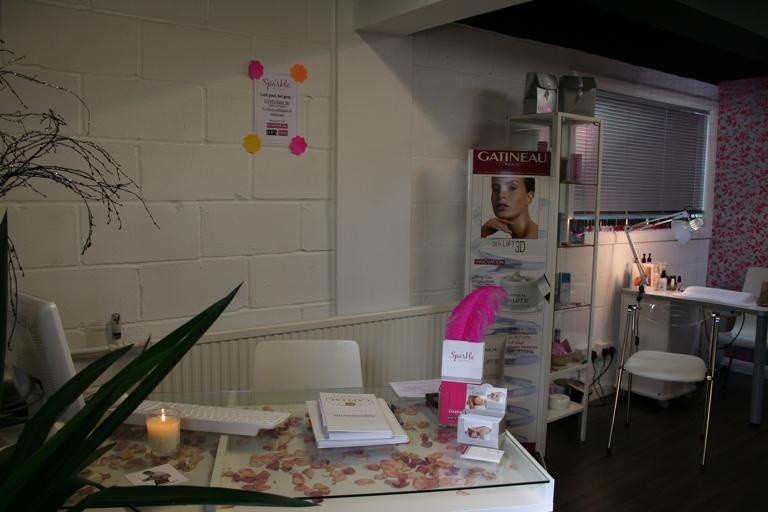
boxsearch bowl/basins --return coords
[549,393,570,411]
[551,353,576,366]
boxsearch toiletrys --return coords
[632,254,654,289]
[658,270,668,291]
[658,270,682,291]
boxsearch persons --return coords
[464,425,491,441]
[467,395,486,410]
[479,175,539,240]
[487,391,504,401]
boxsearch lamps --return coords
[623,208,704,352]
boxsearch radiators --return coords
[71,301,462,404]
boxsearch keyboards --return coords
[108,399,292,436]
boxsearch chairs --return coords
[252,339,364,394]
[607,299,719,465]
[718,266,768,372]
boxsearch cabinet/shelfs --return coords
[503,112,602,456]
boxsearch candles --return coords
[144,407,182,453]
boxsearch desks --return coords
[2,372,555,512]
[617,287,768,424]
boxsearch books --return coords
[305,391,409,448]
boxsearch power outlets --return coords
[574,344,597,364]
[594,338,616,360]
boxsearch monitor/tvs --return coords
[4,289,86,424]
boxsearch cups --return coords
[145,407,181,459]
[756,280,768,307]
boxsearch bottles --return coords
[554,328,560,344]
[658,271,682,292]
[108,311,122,345]
[642,253,646,263]
[583,217,672,234]
[647,254,652,263]
